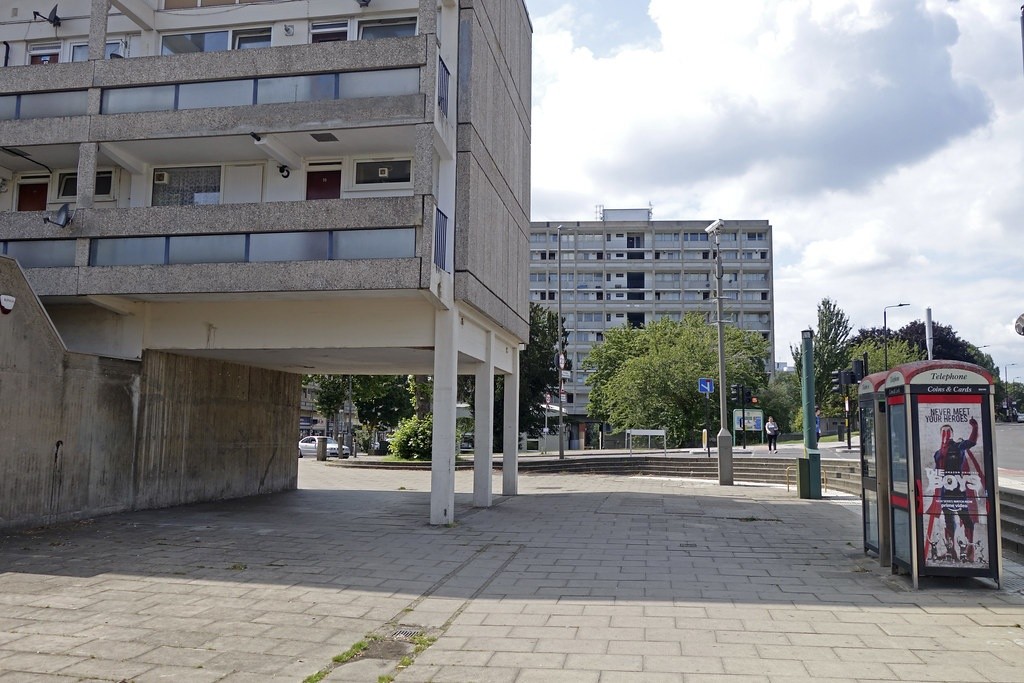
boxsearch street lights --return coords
[884,303,911,372]
[1012,376,1022,400]
[919,336,939,361]
[977,344,991,366]
[557,224,567,458]
[1005,363,1018,408]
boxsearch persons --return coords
[926,418,978,562]
[765,416,778,454]
[815,408,821,447]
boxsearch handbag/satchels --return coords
[774,423,780,436]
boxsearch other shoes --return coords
[774,450,777,454]
[769,450,771,454]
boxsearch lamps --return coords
[33,4,62,27]
[44,201,74,227]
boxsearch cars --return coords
[370,442,381,449]
[1017,414,1024,423]
[298,435,350,459]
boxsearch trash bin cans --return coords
[317,438,327,461]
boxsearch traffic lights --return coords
[830,368,844,397]
[731,384,739,403]
[745,389,752,403]
